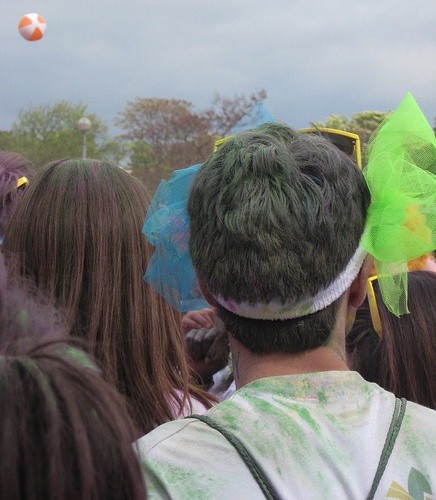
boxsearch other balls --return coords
[18,14,46,41]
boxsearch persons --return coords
[0,152,436,500]
[129,123,436,500]
